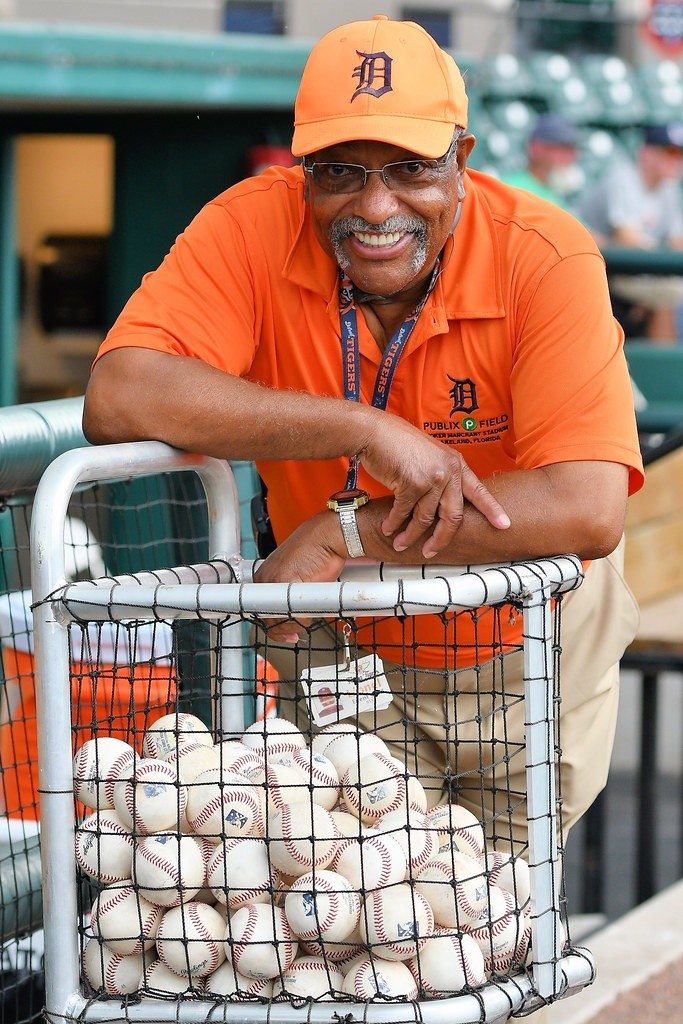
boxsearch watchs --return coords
[327,488,370,559]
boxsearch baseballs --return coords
[74,711,537,1005]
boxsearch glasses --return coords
[303,132,463,195]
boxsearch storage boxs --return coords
[21,441,596,1022]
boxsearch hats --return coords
[646,123,683,148]
[292,14,468,158]
[534,117,584,151]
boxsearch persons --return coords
[82,13,644,897]
[503,111,682,466]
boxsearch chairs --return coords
[439,48,683,203]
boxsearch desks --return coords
[578,587,683,919]
